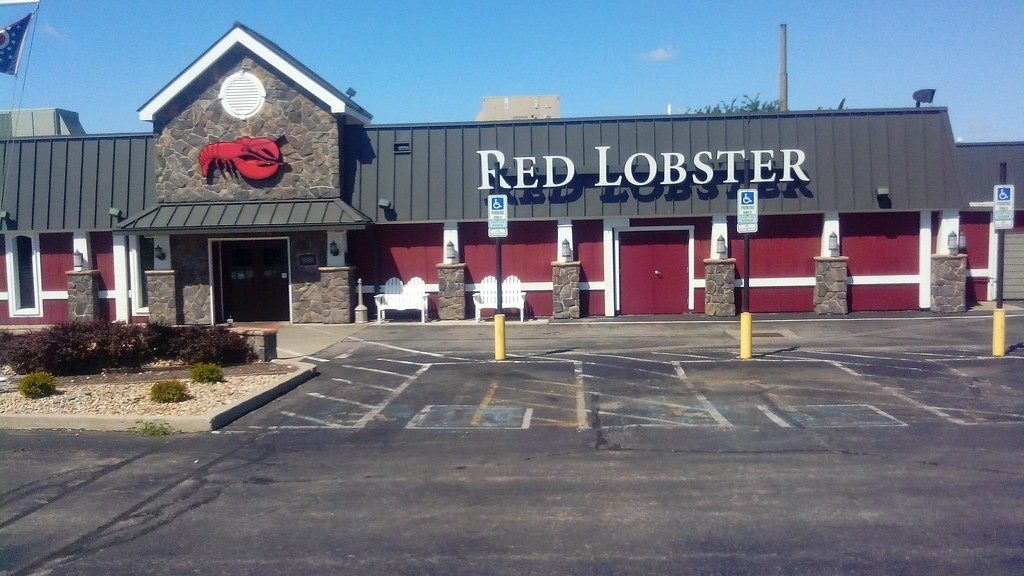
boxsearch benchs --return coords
[373,277,430,323]
[226,326,278,363]
[472,274,526,322]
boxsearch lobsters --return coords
[198,135,283,179]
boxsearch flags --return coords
[0,12,34,76]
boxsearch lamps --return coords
[447,241,455,259]
[562,239,571,256]
[716,235,726,253]
[959,231,966,249]
[829,232,838,250]
[948,232,957,249]
[329,240,340,256]
[154,245,166,260]
[73,249,84,266]
